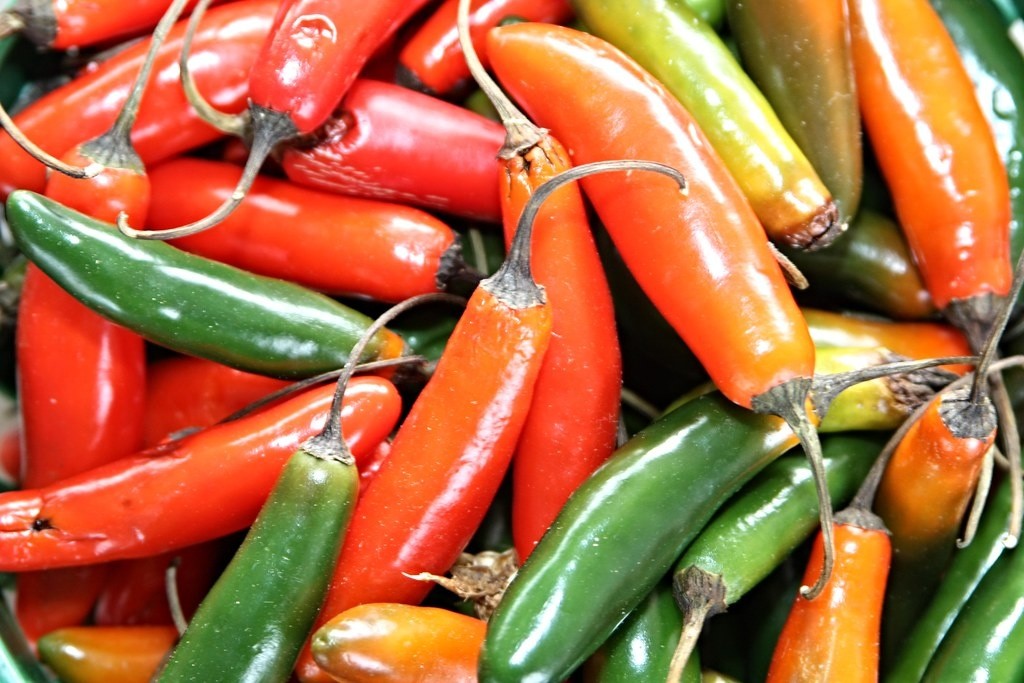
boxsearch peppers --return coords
[0,0,1024,683]
